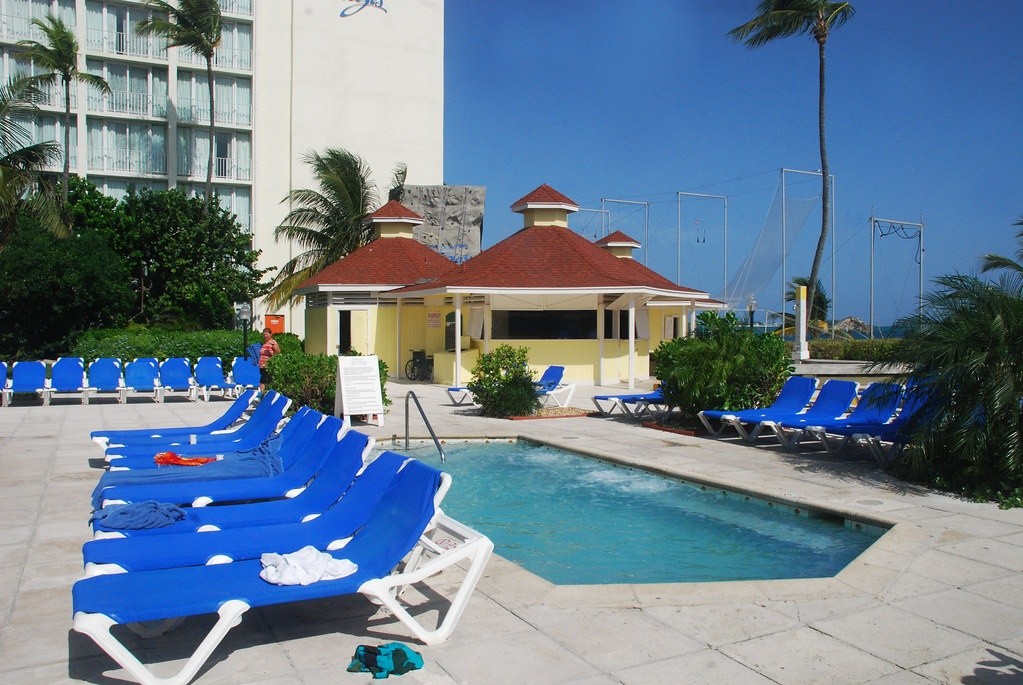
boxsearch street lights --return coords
[746,293,757,333]
[238,302,253,361]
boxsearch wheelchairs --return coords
[405,349,434,382]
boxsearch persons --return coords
[258,327,280,391]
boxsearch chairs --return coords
[0,358,574,685]
[591,381,672,421]
[697,376,935,465]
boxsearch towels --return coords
[259,545,359,587]
[87,499,188,531]
